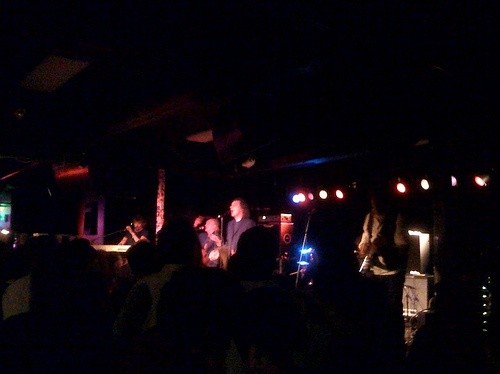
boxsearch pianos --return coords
[91,243,131,253]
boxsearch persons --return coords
[0,214,500,374]
[356,190,411,346]
[223,196,256,260]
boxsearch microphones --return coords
[215,211,231,219]
[307,207,316,217]
